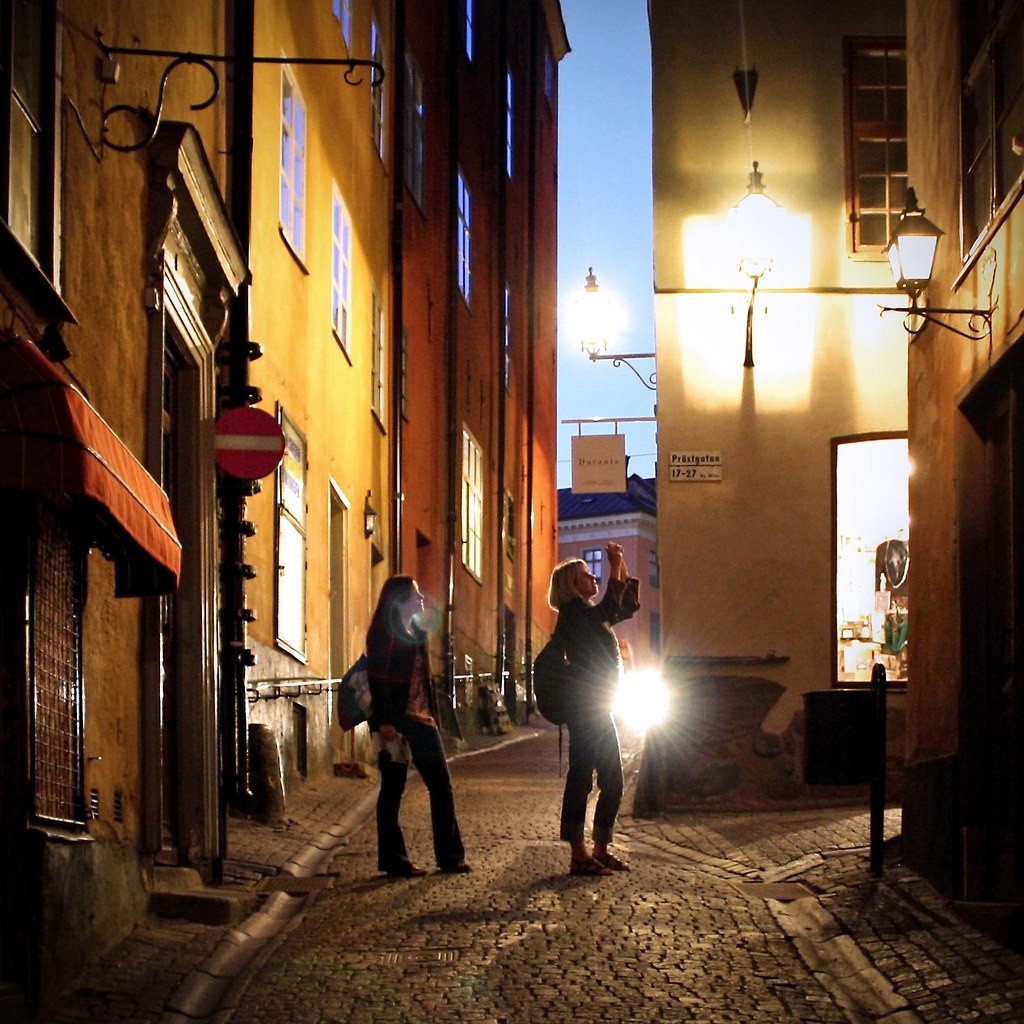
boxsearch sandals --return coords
[570,858,615,876]
[592,853,631,871]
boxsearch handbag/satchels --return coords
[337,653,374,732]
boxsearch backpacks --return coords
[533,639,577,726]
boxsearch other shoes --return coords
[387,865,428,877]
[436,864,474,873]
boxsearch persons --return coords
[547,541,641,876]
[366,574,475,879]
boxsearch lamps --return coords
[578,267,657,390]
[875,187,993,341]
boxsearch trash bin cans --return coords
[799,688,875,787]
